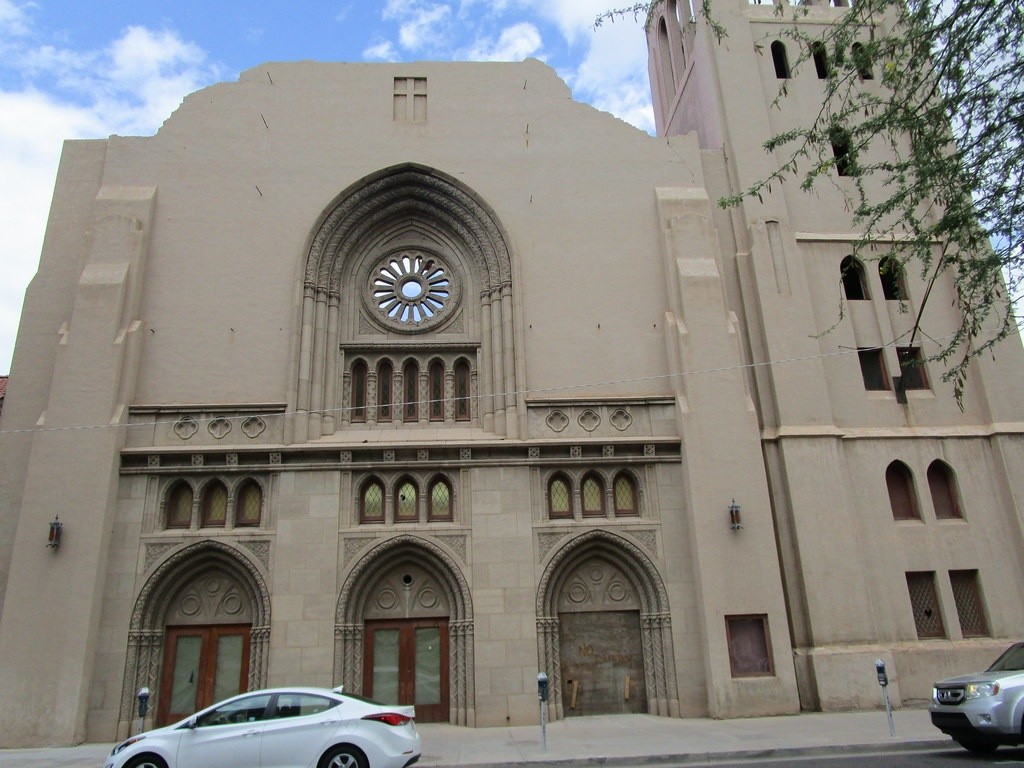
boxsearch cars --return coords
[102,685,421,768]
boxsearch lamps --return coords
[46,514,63,548]
[728,498,744,530]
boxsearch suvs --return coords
[925,642,1024,759]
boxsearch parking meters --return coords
[875,659,895,738]
[138,684,152,737]
[537,672,549,756]
